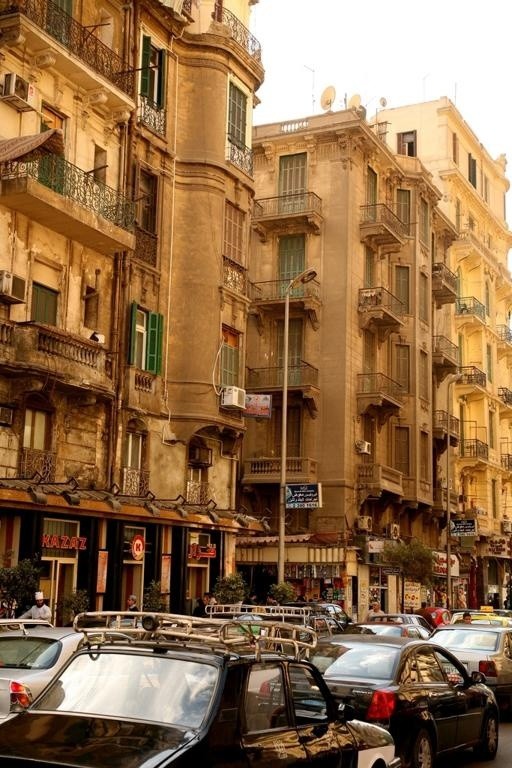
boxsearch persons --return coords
[462,613,472,624]
[364,602,387,624]
[124,593,139,618]
[17,590,52,624]
[194,591,326,619]
[414,601,437,627]
[445,672,461,683]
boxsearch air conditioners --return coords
[355,440,372,456]
[1,269,28,304]
[503,521,512,532]
[220,385,246,411]
[357,515,373,532]
[0,72,38,114]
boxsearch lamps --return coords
[0,470,273,532]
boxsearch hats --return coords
[34,592,44,600]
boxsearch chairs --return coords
[84,670,272,731]
[311,654,393,678]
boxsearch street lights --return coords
[277,268,319,582]
[446,373,466,607]
